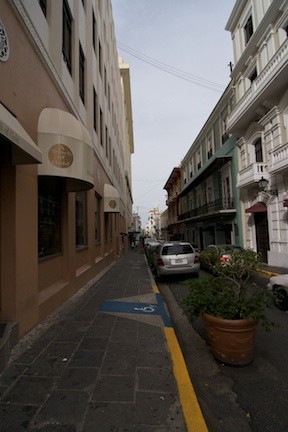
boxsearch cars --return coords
[144,238,170,266]
[201,245,246,276]
[151,240,200,280]
[266,273,288,310]
[191,243,200,253]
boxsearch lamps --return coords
[257,176,278,196]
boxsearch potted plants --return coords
[174,247,285,368]
